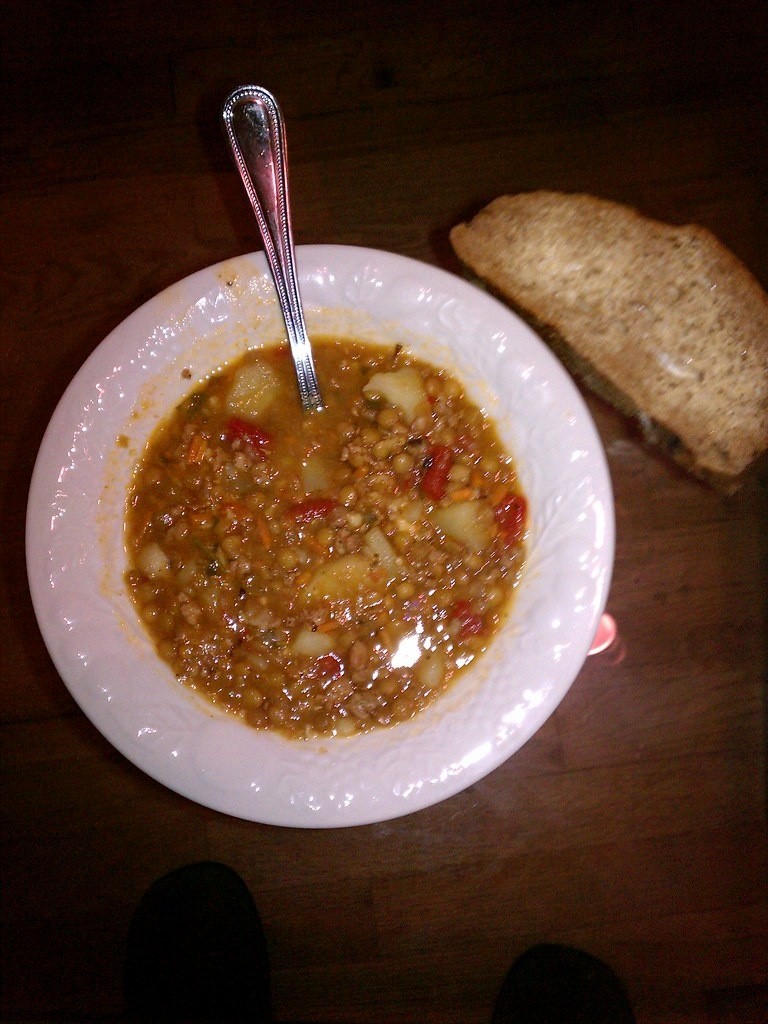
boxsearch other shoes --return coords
[121,862,274,1024]
[488,943,638,1024]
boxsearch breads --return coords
[448,189,768,496]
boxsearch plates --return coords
[25,245,615,829]
